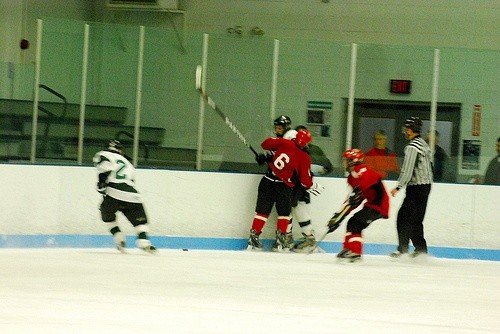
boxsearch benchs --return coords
[0,99,197,172]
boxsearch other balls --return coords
[182,248,189,251]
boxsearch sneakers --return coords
[272,230,291,252]
[247,229,264,250]
[145,245,157,255]
[335,248,362,265]
[285,232,297,250]
[293,232,316,253]
[117,241,127,255]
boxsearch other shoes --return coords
[392,250,409,258]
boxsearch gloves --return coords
[328,212,346,232]
[348,186,363,209]
[306,187,321,197]
[256,151,276,166]
[97,182,106,195]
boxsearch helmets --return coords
[283,130,298,140]
[404,116,422,133]
[275,115,291,127]
[343,149,364,172]
[105,139,124,150]
[295,129,312,147]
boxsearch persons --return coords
[328,149,389,263]
[425,130,446,183]
[255,115,313,253]
[365,131,398,179]
[391,118,433,257]
[93,140,156,254]
[294,125,334,177]
[483,137,500,185]
[247,130,316,253]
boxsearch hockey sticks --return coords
[195,65,258,158]
[294,204,352,254]
